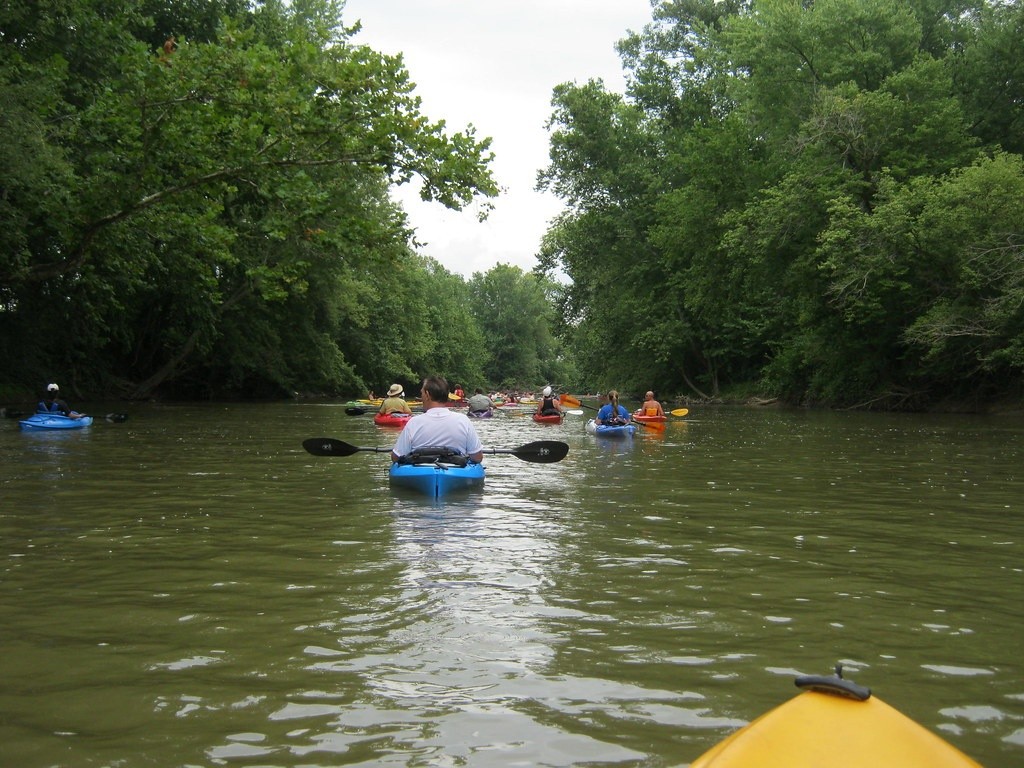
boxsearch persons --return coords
[379,383,412,416]
[491,391,533,398]
[467,388,497,411]
[37,383,86,418]
[391,377,483,463]
[537,386,563,415]
[454,384,464,397]
[637,391,664,416]
[368,390,374,400]
[595,390,631,425]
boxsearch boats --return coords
[374,412,413,428]
[532,411,565,424]
[584,419,637,438]
[386,445,487,504]
[19,410,94,432]
[631,412,668,425]
[468,412,492,418]
[356,397,542,412]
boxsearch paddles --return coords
[3,408,129,423]
[559,394,689,433]
[448,392,508,413]
[344,407,426,415]
[302,437,569,464]
[518,409,584,416]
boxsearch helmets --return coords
[47,384,59,392]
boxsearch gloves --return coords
[80,413,86,418]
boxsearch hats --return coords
[543,386,551,397]
[387,384,403,396]
